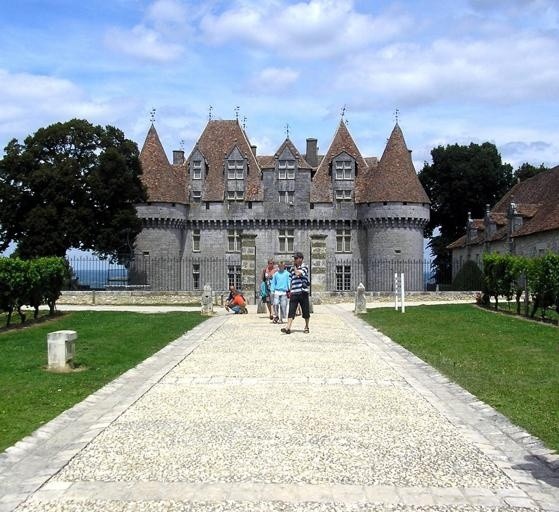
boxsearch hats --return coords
[292,252,303,259]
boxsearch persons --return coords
[228,292,248,315]
[226,285,246,314]
[261,252,311,334]
